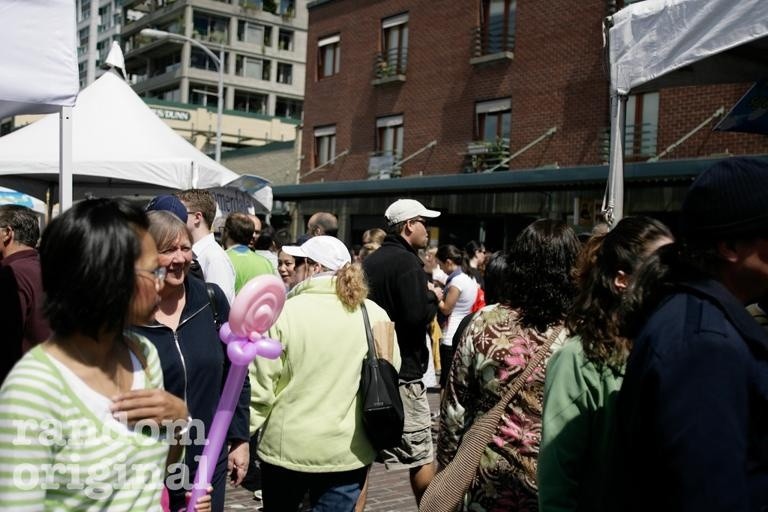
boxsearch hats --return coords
[384,199,442,226]
[145,194,187,225]
[281,235,352,273]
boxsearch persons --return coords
[0,156,768,512]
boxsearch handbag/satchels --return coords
[419,407,504,512]
[362,357,405,453]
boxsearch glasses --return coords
[135,264,168,282]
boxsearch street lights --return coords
[137,25,225,166]
